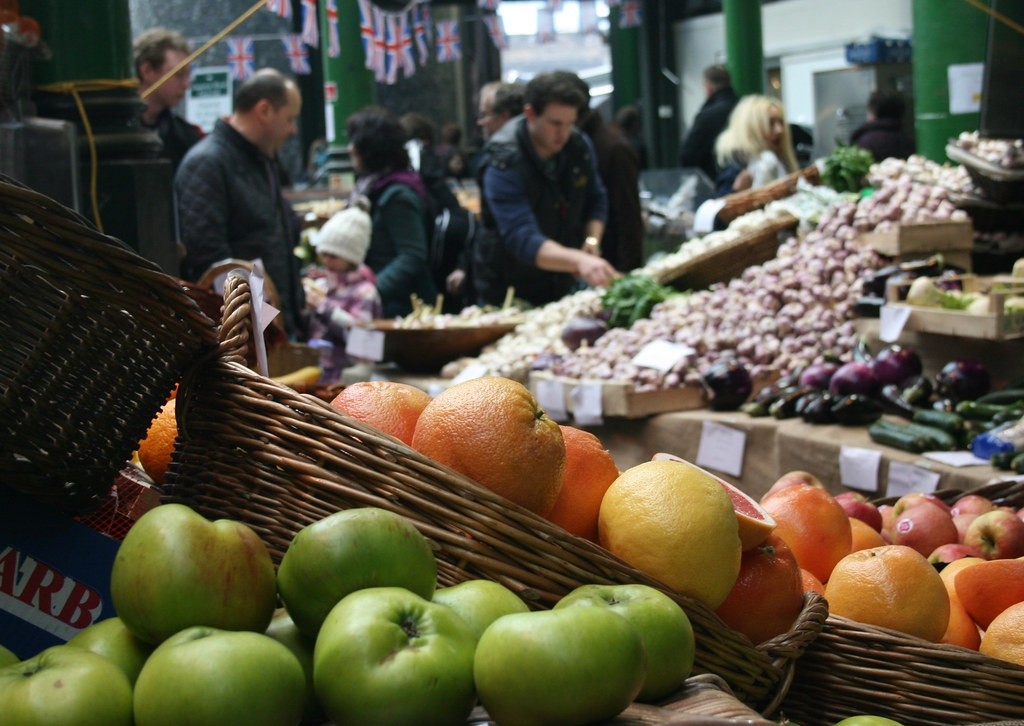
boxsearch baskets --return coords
[778,608,1024,726]
[159,276,827,715]
[712,165,821,220]
[0,172,213,538]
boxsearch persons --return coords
[133,26,207,164]
[302,206,384,384]
[345,104,480,322]
[170,69,311,344]
[473,70,647,306]
[848,87,915,164]
[680,64,815,200]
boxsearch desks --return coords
[373,344,1024,511]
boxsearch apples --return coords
[835,715,905,726]
[760,471,1024,563]
[0,504,692,726]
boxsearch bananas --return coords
[269,365,322,388]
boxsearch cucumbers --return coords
[869,401,1024,453]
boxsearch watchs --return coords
[584,237,599,247]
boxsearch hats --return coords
[311,195,372,266]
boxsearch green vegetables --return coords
[822,137,874,193]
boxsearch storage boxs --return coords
[525,220,1024,422]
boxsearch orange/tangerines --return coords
[130,383,178,486]
[331,379,807,644]
[757,484,1024,666]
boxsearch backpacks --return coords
[427,206,479,301]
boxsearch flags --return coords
[358,1,435,84]
[226,39,255,80]
[477,0,643,49]
[280,36,312,74]
[434,21,463,63]
[326,0,341,59]
[267,0,294,21]
[299,0,320,49]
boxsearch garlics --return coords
[957,131,1024,167]
[391,154,979,391]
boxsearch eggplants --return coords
[739,255,990,425]
[702,363,753,412]
